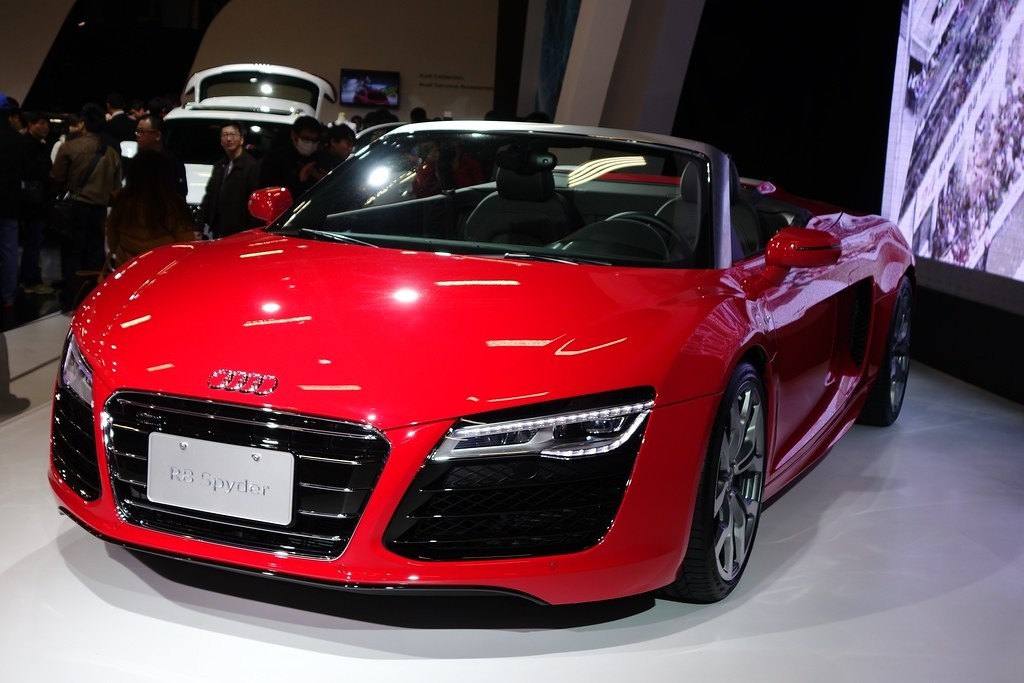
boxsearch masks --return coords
[294,135,318,156]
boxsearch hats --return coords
[0,94,10,109]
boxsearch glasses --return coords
[296,133,320,144]
[135,129,159,138]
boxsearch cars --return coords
[105,62,491,225]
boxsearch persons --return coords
[0,90,554,422]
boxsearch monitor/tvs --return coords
[340,68,400,109]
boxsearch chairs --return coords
[646,157,757,256]
[458,151,573,239]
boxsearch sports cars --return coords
[48,121,918,607]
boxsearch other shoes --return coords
[0,397,30,415]
[4,299,13,307]
[20,280,56,294]
